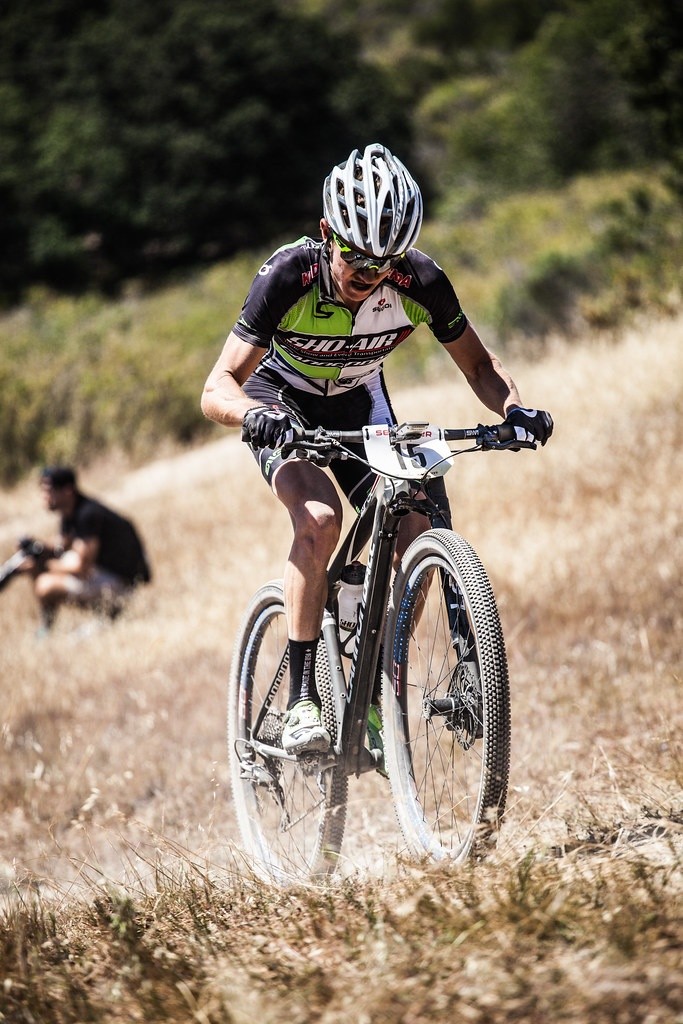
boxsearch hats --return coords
[40,466,75,489]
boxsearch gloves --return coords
[242,405,298,460]
[499,404,554,453]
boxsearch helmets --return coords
[323,143,424,260]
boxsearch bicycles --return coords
[226,409,541,886]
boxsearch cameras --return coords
[0,537,44,590]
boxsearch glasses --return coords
[338,250,406,272]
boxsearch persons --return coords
[200,143,555,779]
[10,467,151,621]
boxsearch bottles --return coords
[337,561,367,654]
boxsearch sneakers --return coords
[282,701,331,756]
[366,703,390,782]
